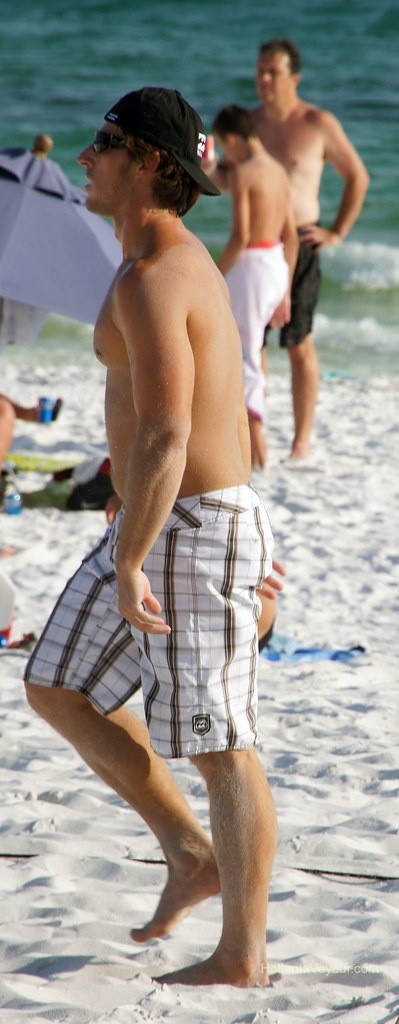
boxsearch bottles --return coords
[3,476,31,538]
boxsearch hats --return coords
[93,129,128,154]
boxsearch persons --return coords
[0,395,19,465]
[213,103,300,471]
[105,492,276,652]
[21,88,284,991]
[202,36,370,461]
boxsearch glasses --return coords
[104,87,222,196]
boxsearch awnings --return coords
[0,135,125,325]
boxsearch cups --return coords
[201,134,215,165]
[38,395,54,426]
